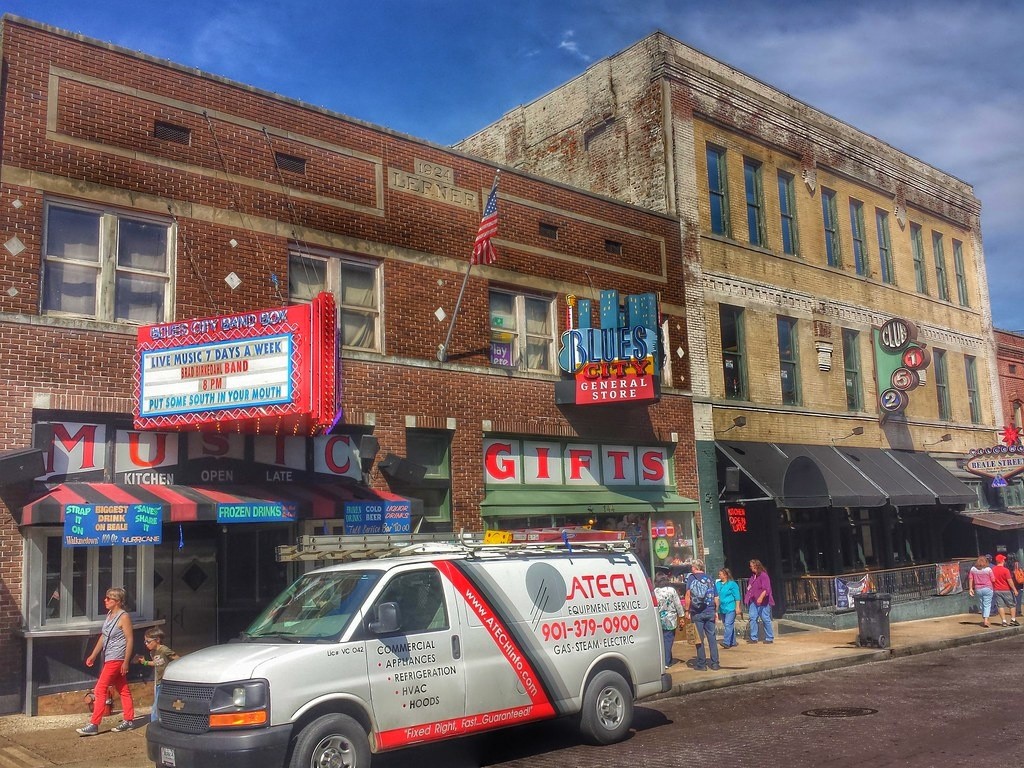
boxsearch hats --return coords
[986,554,992,559]
[996,555,1006,562]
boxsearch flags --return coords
[470,186,498,265]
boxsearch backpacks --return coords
[689,573,711,610]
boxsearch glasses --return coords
[748,565,754,567]
[106,596,118,600]
[144,639,157,643]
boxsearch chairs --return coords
[333,586,441,633]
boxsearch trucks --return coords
[146,530,672,768]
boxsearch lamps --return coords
[923,434,952,446]
[834,427,863,440]
[715,416,746,433]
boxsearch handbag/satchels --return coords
[1013,568,1024,583]
[685,622,701,645]
[100,651,105,664]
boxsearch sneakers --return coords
[111,720,134,733]
[76,723,98,735]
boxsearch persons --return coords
[715,568,740,649]
[653,571,685,668]
[685,559,719,671]
[744,560,775,644]
[138,628,180,722]
[969,554,1024,628]
[76,588,135,735]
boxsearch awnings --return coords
[717,440,978,505]
[19,481,424,526]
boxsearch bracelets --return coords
[144,661,147,666]
[686,610,689,612]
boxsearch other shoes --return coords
[720,642,730,648]
[764,639,773,644]
[712,662,720,669]
[732,641,739,646]
[1009,619,1020,626]
[984,623,990,627]
[1002,622,1008,626]
[696,663,707,670]
[1016,611,1023,616]
[748,639,759,644]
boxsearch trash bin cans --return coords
[851,593,892,649]
